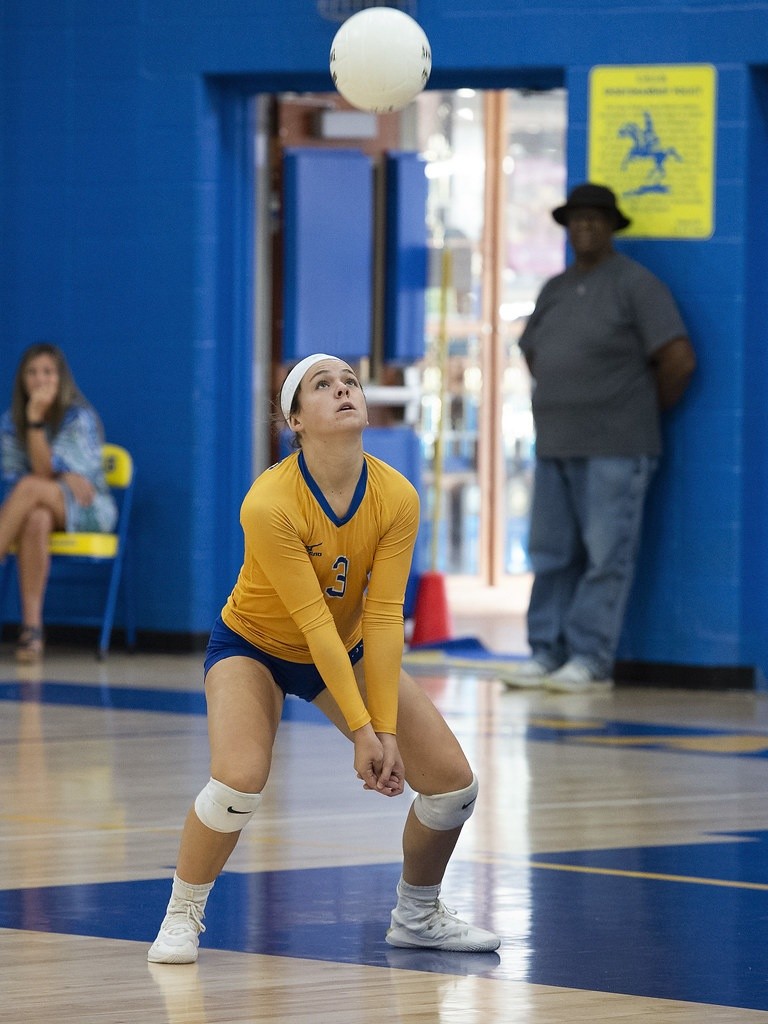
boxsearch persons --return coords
[503,183,698,693]
[144,353,503,965]
[0,340,117,663]
[435,362,475,568]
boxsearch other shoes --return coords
[17,620,42,666]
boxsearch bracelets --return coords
[27,422,49,428]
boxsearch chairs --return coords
[6,442,137,658]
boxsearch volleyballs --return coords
[331,6,436,113]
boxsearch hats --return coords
[552,181,631,232]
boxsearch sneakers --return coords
[385,888,501,953]
[541,658,614,692]
[497,661,551,688]
[387,943,500,982]
[146,891,206,963]
[151,964,202,996]
